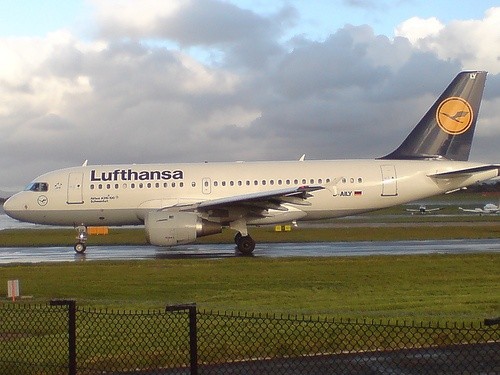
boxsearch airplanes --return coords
[2,70,500,255]
[458,203,499,216]
[405,205,444,215]
